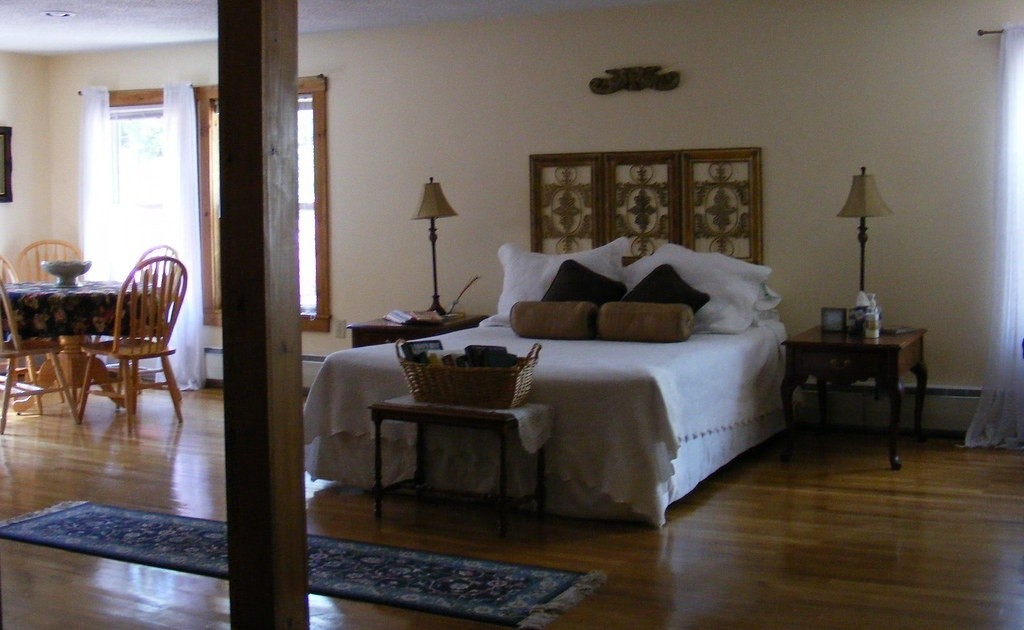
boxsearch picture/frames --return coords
[0,126,14,203]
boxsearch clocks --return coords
[820,307,848,334]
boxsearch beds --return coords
[304,317,787,529]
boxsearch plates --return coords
[41,260,92,287]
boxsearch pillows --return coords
[620,264,710,315]
[497,235,629,315]
[598,302,695,343]
[508,301,598,341]
[541,258,628,308]
[615,243,783,334]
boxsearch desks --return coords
[0,281,160,414]
[366,394,554,540]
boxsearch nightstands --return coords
[780,325,927,470]
[346,312,491,349]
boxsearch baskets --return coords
[394,338,543,409]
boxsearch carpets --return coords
[0,501,608,630]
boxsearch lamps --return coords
[410,176,459,316]
[835,167,895,292]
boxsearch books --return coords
[400,340,518,368]
[382,309,442,324]
[882,325,917,335]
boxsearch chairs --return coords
[0,239,187,436]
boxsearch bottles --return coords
[854,290,870,334]
[866,294,877,308]
[865,307,879,338]
